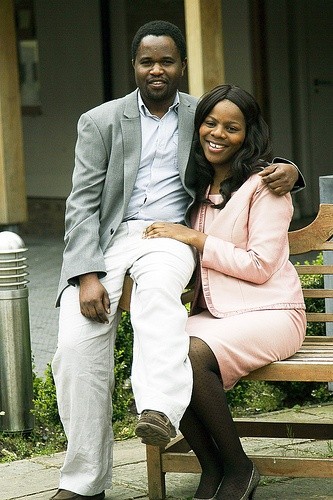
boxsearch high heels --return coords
[194,475,224,500]
[239,461,260,500]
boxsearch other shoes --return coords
[50,489,106,500]
[135,410,172,450]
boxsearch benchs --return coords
[116,204,333,500]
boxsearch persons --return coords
[49,20,308,499]
[141,84,306,500]
